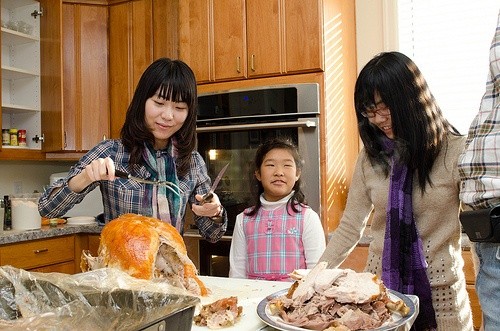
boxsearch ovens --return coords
[182,83,321,278]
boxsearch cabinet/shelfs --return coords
[153,0,357,85]
[48,0,153,160]
[0,234,75,275]
[0,0,67,152]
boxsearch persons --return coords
[458,7,500,331]
[316,52,477,331]
[228,138,326,281]
[37,58,227,243]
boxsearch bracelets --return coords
[209,205,224,220]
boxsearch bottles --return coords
[0,195,11,231]
[2,129,28,147]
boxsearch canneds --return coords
[2,128,27,146]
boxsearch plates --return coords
[256,287,416,330]
[41,217,98,226]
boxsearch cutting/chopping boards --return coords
[189,275,293,330]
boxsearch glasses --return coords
[361,107,391,118]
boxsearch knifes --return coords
[104,168,158,185]
[199,163,229,206]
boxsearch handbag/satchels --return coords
[458,205,500,242]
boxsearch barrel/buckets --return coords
[8,192,44,230]
